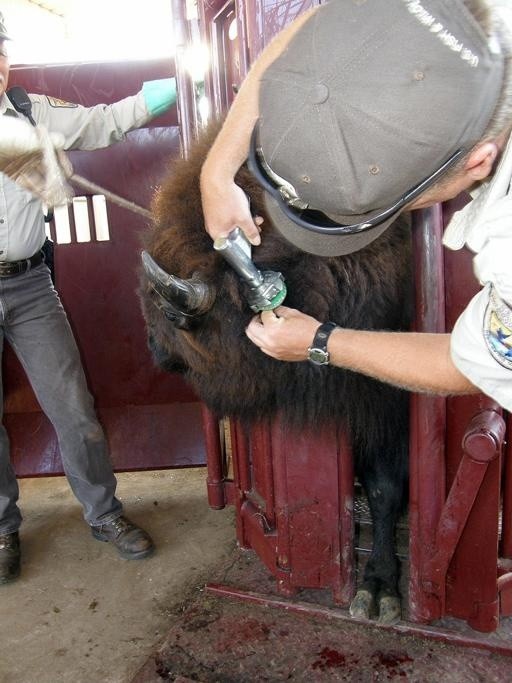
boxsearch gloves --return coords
[141,77,206,113]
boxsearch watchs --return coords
[307,316,334,366]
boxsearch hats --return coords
[0,7,16,41]
[241,0,503,259]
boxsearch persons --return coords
[1,5,204,578]
[196,0,512,416]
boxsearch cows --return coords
[133,103,418,628]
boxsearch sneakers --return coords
[90,515,155,560]
[0,532,23,581]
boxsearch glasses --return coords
[247,116,466,235]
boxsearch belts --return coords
[0,251,44,277]
